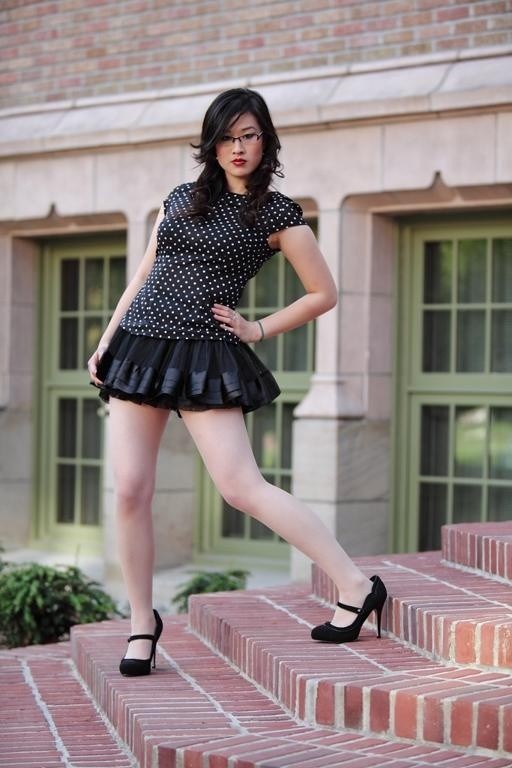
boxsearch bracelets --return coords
[255,319,264,342]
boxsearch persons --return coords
[86,87,387,678]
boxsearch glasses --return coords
[220,132,263,146]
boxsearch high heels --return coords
[119,609,163,677]
[311,575,387,643]
